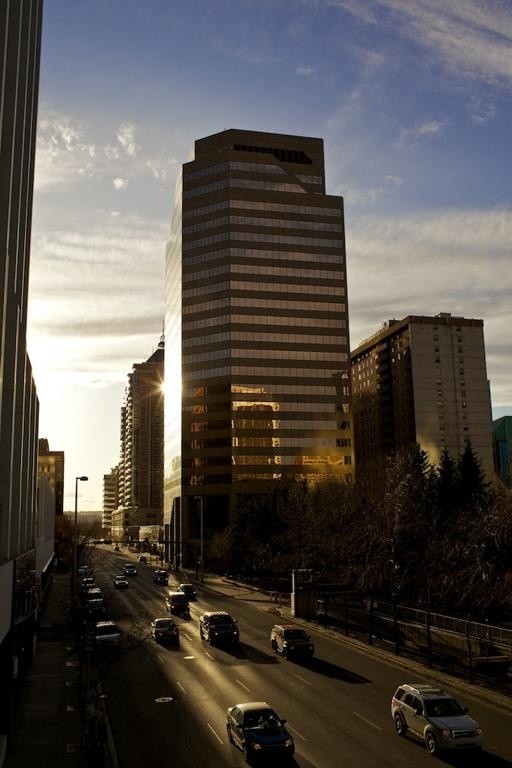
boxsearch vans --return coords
[138,555,146,564]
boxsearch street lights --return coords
[72,477,88,602]
[195,496,204,583]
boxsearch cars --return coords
[166,583,197,620]
[78,563,137,645]
[153,571,170,585]
[226,702,295,762]
[150,617,179,643]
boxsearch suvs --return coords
[270,624,314,660]
[199,612,239,645]
[390,682,484,755]
